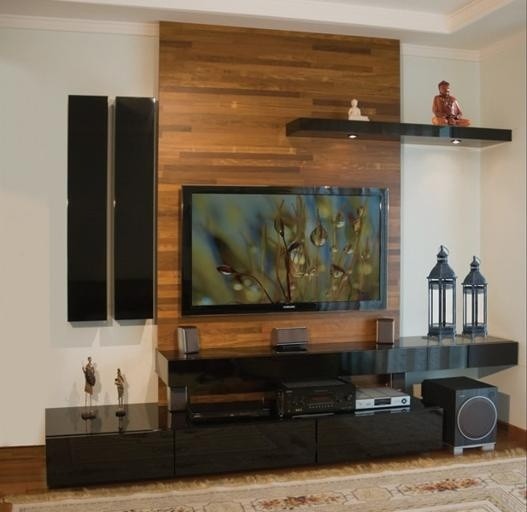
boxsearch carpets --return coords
[0,446,526,512]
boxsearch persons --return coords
[432,81,471,127]
[349,98,361,116]
[83,356,95,408]
[115,369,124,408]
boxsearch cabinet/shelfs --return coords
[45,335,519,490]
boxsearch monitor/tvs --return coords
[181,185,389,318]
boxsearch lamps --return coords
[461,254,486,336]
[428,246,455,339]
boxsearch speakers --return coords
[67,95,109,322]
[421,376,498,456]
[177,326,199,354]
[114,96,154,320]
[375,318,395,345]
[271,326,309,350]
